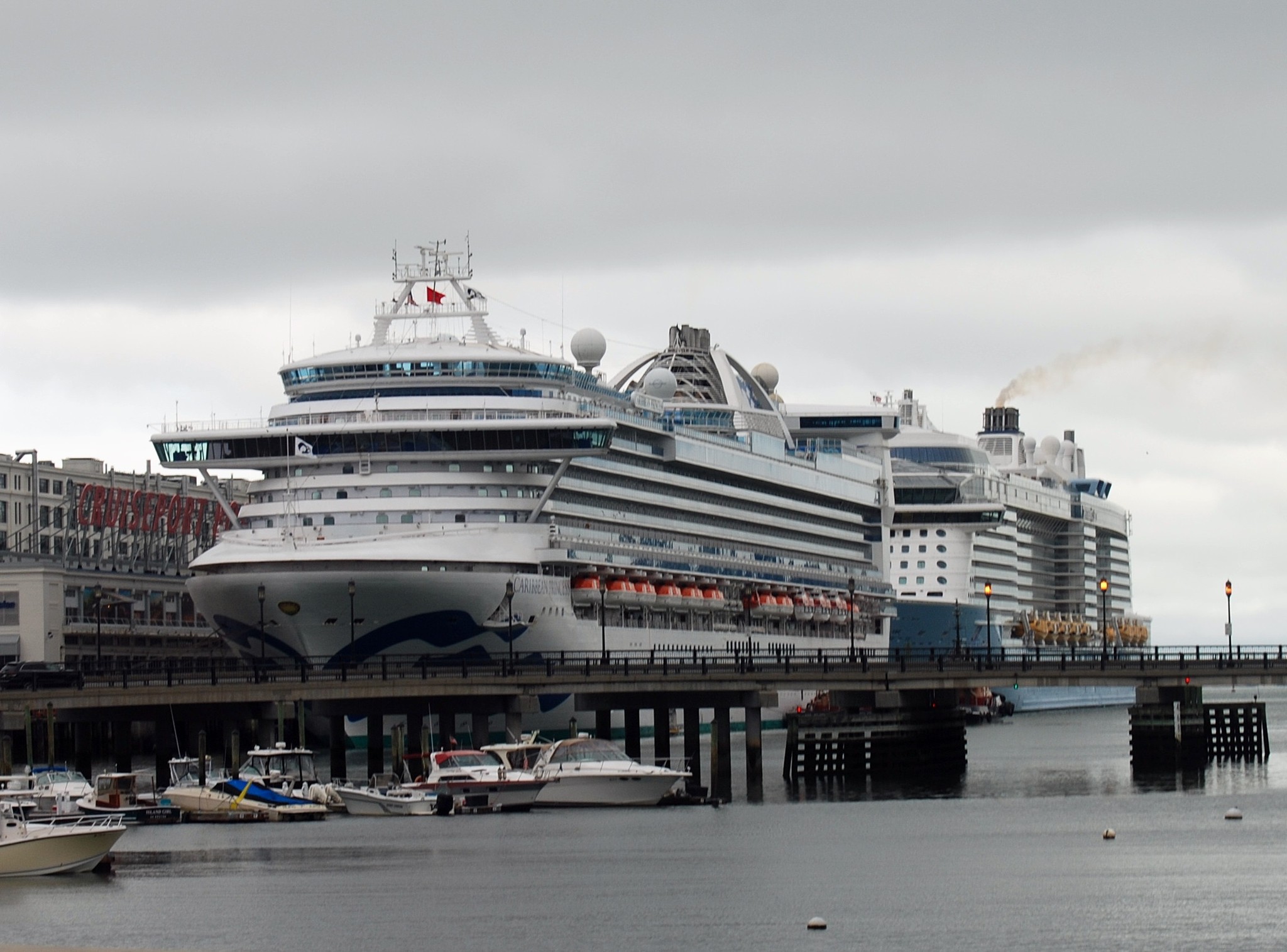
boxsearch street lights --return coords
[847,575,857,664]
[745,579,754,664]
[1225,579,1233,661]
[598,573,609,665]
[983,579,993,661]
[348,576,357,661]
[257,580,266,664]
[1098,575,1109,665]
[94,580,105,675]
[505,579,516,665]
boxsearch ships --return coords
[151,225,1140,760]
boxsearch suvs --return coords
[0,661,87,692]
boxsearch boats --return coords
[572,575,727,612]
[1013,612,1151,647]
[780,686,1018,731]
[0,793,126,882]
[1,728,691,824]
[748,586,858,627]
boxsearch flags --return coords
[407,290,419,307]
[872,395,881,403]
[294,436,315,458]
[449,736,458,744]
[426,287,445,304]
